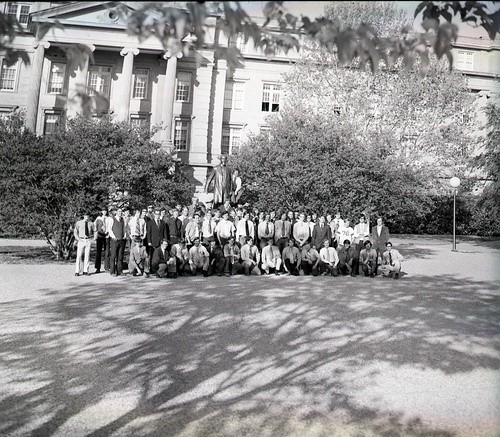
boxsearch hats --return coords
[207,236,215,242]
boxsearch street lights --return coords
[450,177,461,252]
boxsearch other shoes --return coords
[394,273,399,280]
[388,273,393,278]
[75,273,79,276]
[95,270,100,273]
[83,272,90,276]
[350,271,357,277]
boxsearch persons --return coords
[151,238,178,279]
[338,239,359,277]
[359,240,377,278]
[170,237,189,279]
[188,236,210,277]
[128,237,151,278]
[73,203,390,277]
[232,168,246,202]
[217,200,233,216]
[377,242,404,279]
[205,154,233,207]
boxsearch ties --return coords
[289,248,294,264]
[84,221,89,236]
[281,222,285,236]
[265,222,269,235]
[308,227,311,237]
[157,220,160,228]
[230,247,234,264]
[208,220,212,234]
[269,246,274,260]
[135,218,141,235]
[245,220,249,236]
[307,252,312,264]
[249,246,254,259]
[326,250,330,261]
[389,252,393,265]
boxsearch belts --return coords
[80,237,91,239]
